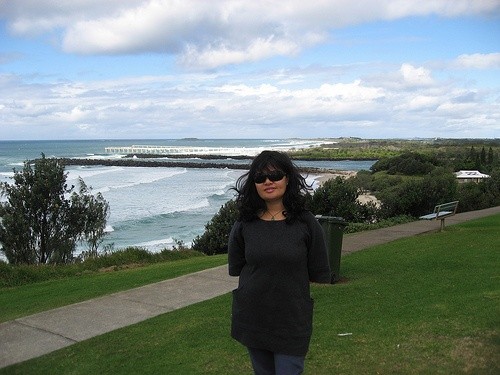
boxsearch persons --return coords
[227,150,332,375]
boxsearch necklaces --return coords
[266,207,285,220]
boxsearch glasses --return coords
[255,170,287,184]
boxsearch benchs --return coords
[419,201,459,232]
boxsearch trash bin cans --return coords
[314,212,348,283]
